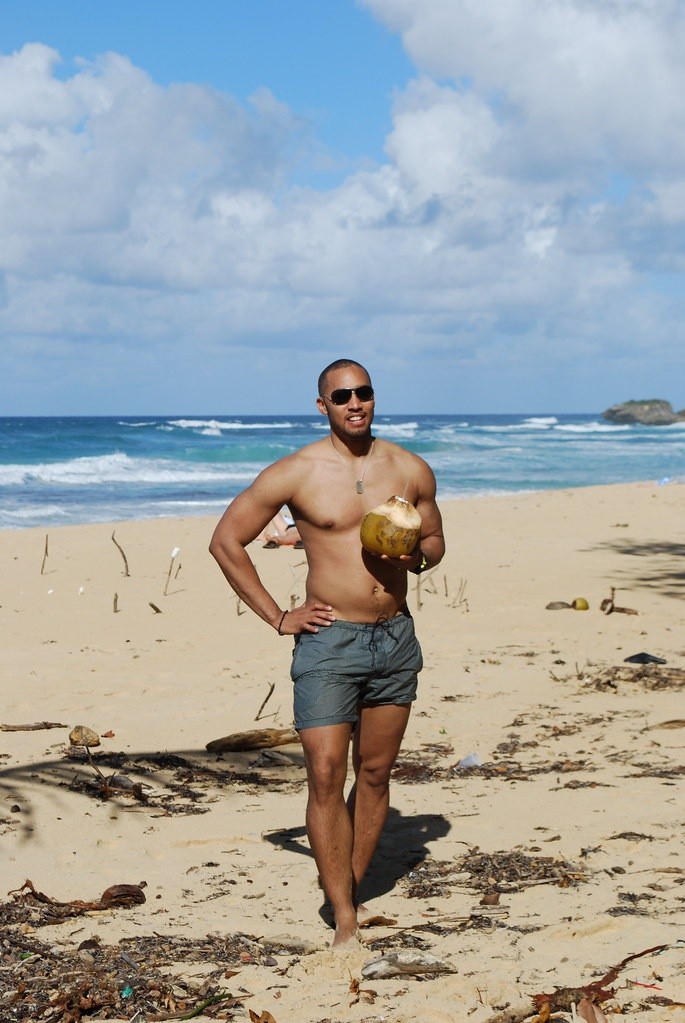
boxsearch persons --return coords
[264,512,301,546]
[208,359,445,947]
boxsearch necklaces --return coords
[329,437,374,493]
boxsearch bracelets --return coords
[278,609,288,636]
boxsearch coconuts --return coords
[571,598,589,609]
[360,493,420,558]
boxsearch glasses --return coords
[321,386,374,405]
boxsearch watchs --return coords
[415,553,427,574]
